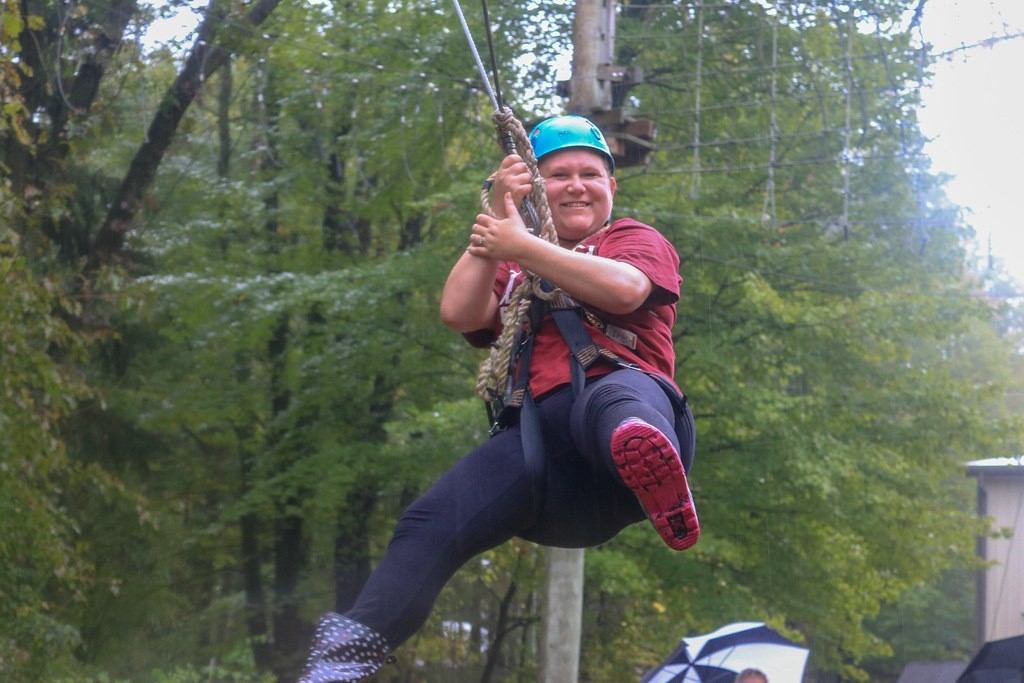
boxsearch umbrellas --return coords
[898,632,1024,683]
[643,620,811,683]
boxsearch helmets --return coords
[529,114,615,175]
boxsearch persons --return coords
[736,667,768,683]
[294,113,701,683]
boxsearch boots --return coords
[611,418,699,550]
[299,611,391,683]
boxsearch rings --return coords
[477,235,484,246]
